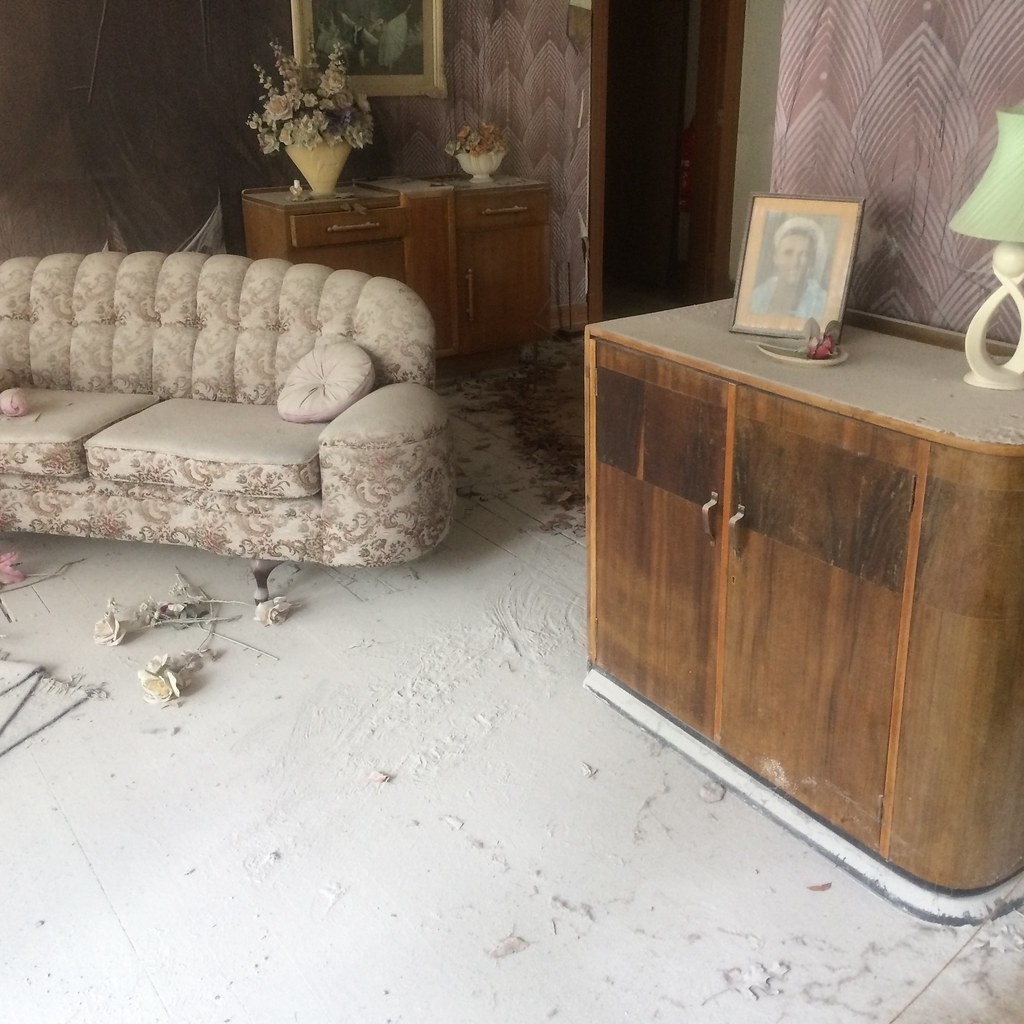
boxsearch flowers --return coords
[244,37,375,154]
[444,120,507,157]
[745,318,842,360]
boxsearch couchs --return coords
[0,251,456,606]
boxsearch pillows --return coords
[275,340,374,423]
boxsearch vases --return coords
[284,136,352,193]
[455,150,507,185]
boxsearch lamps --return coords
[947,108,1024,392]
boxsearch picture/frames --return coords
[290,0,447,99]
[729,192,866,345]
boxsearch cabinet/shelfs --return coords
[241,173,552,375]
[584,294,1024,928]
